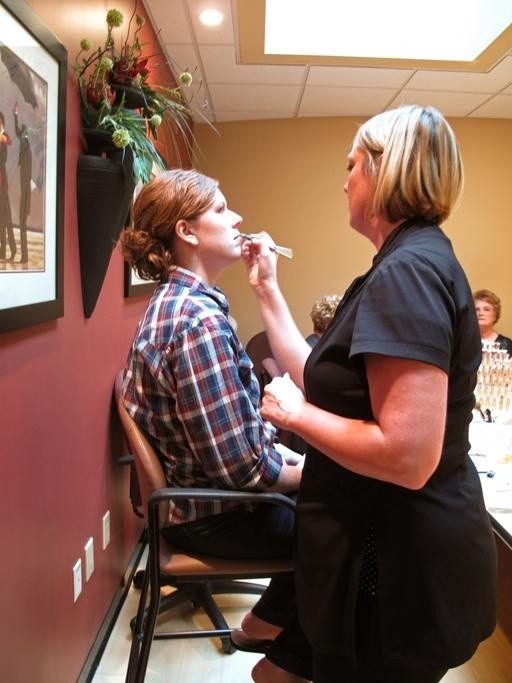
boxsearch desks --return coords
[461,419,512,642]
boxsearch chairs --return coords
[107,366,301,682]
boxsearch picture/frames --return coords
[0,0,69,332]
[124,145,166,298]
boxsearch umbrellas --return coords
[0,45,39,111]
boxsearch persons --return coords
[12,101,32,263]
[118,169,306,641]
[290,295,342,456]
[0,112,16,261]
[473,289,512,360]
[241,104,498,683]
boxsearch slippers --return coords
[229,608,278,653]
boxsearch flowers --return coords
[68,0,223,190]
